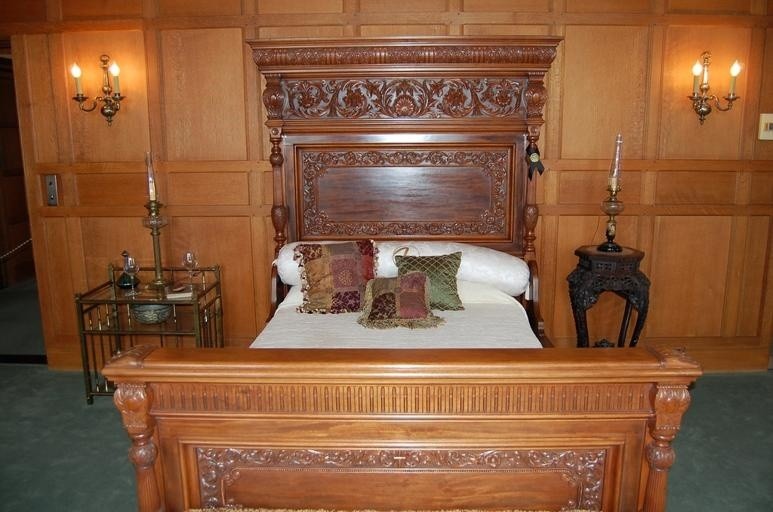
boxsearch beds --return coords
[102,252,707,512]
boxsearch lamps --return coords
[141,148,172,291]
[70,53,126,128]
[685,52,742,126]
[596,134,626,251]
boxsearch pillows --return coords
[294,239,380,315]
[393,251,465,311]
[356,271,447,329]
[272,240,530,300]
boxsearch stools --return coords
[567,245,651,349]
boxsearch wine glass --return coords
[182,248,198,287]
[123,255,140,297]
